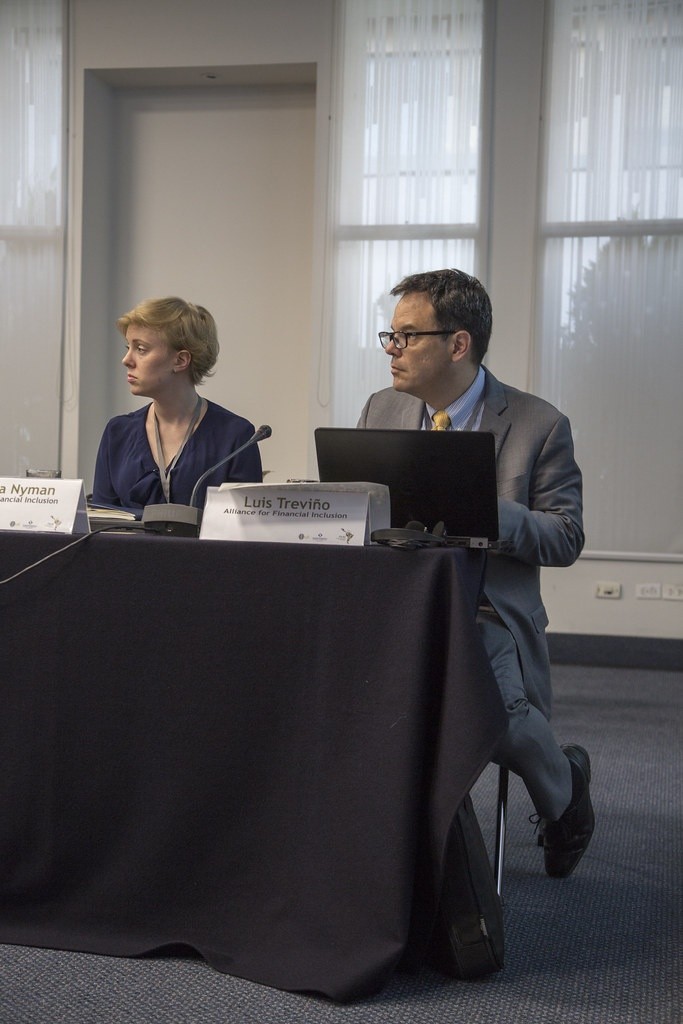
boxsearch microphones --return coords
[142,424,272,538]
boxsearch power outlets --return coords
[636,583,663,599]
[662,584,683,601]
[596,582,621,599]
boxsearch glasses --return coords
[378,330,455,349]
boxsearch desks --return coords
[2,527,484,946]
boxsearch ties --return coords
[433,410,454,432]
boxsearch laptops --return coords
[314,428,514,550]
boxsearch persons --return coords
[354,269,596,880]
[91,295,261,514]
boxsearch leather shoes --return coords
[542,745,595,879]
